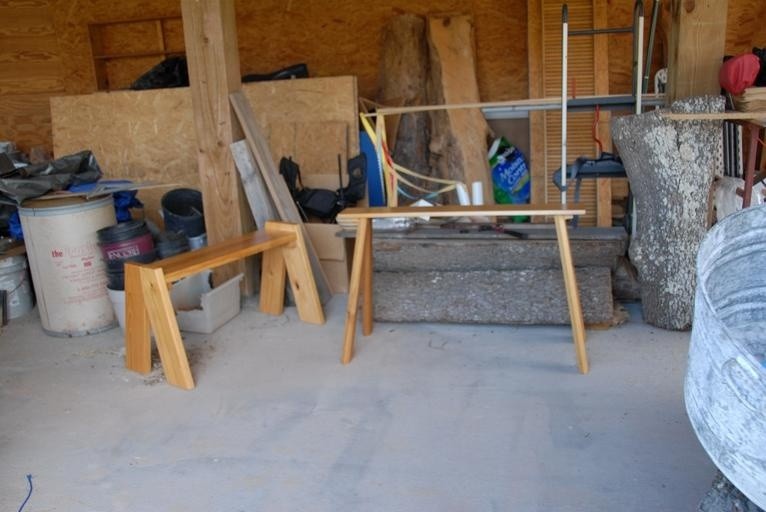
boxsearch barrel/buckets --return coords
[0,191,156,337]
[160,188,203,232]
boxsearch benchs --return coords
[334,203,588,373]
[123,220,326,390]
